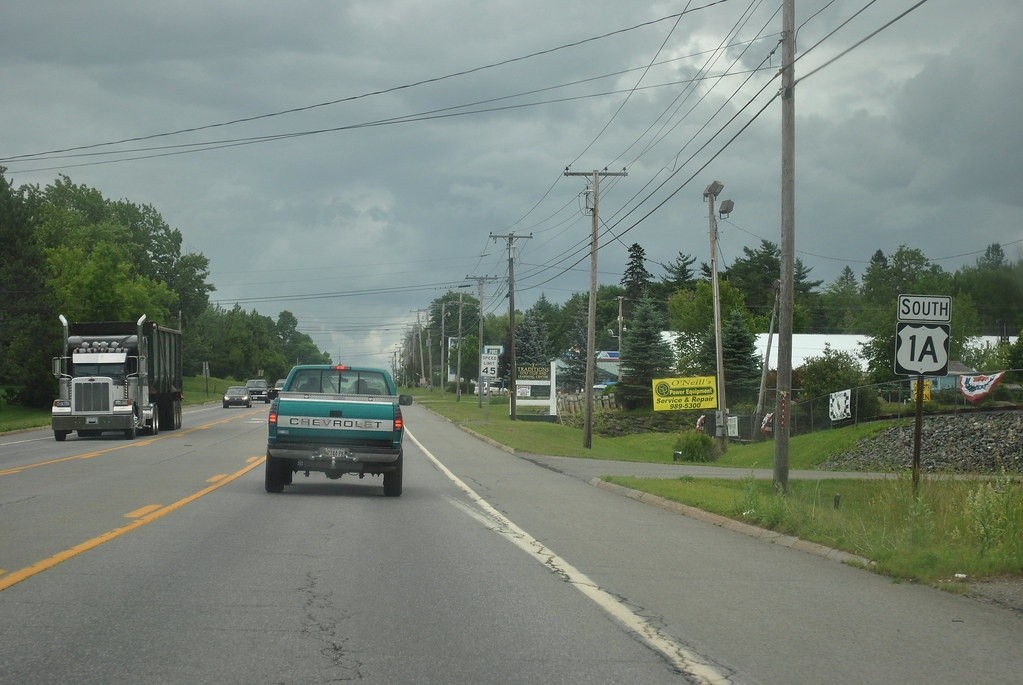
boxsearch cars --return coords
[222,386,252,408]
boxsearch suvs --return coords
[244,379,270,404]
[474,381,508,397]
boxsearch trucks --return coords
[51,309,183,441]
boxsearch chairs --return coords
[298,383,324,392]
[357,389,380,395]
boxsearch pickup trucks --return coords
[265,364,413,497]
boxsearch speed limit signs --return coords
[481,353,497,376]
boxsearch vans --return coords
[275,378,287,390]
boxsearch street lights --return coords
[440,311,451,397]
[703,180,734,453]
